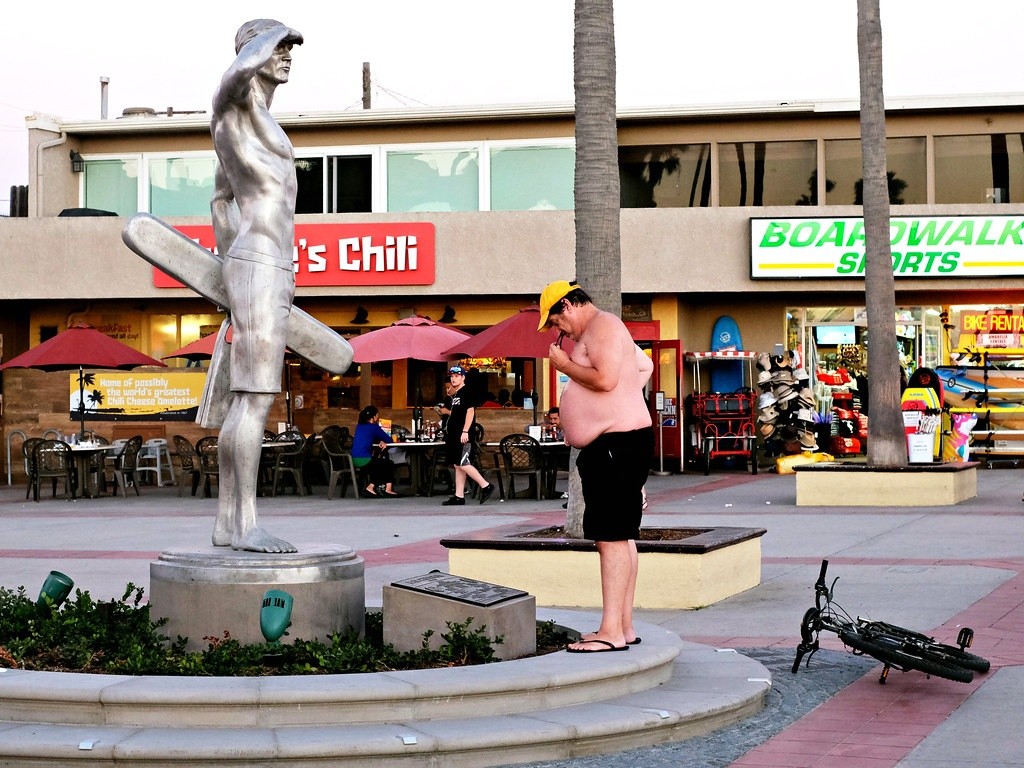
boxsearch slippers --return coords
[583,633,641,644]
[566,641,629,652]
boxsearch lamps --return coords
[259,590,293,654]
[37,571,74,607]
[69,148,85,172]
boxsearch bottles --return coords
[542,426,545,442]
[552,427,558,440]
[426,419,436,438]
[71,433,76,445]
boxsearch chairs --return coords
[23,425,569,503]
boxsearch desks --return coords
[373,441,446,496]
[41,444,120,499]
[481,441,566,500]
[208,442,296,496]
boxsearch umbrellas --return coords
[347,313,472,443]
[160,331,299,360]
[439,302,576,425]
[0,320,168,441]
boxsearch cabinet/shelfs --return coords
[982,352,1024,467]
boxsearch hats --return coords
[446,366,466,376]
[537,280,582,333]
[756,350,819,453]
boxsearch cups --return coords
[399,429,405,442]
[392,434,398,443]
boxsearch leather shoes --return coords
[442,494,466,505]
[481,484,495,503]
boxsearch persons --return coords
[210,19,303,554]
[899,358,907,396]
[857,359,868,454]
[438,366,495,506]
[536,280,654,653]
[524,407,569,469]
[352,405,398,496]
[481,389,523,407]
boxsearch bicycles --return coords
[791,559,990,686]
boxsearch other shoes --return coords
[384,491,400,497]
[362,489,383,498]
